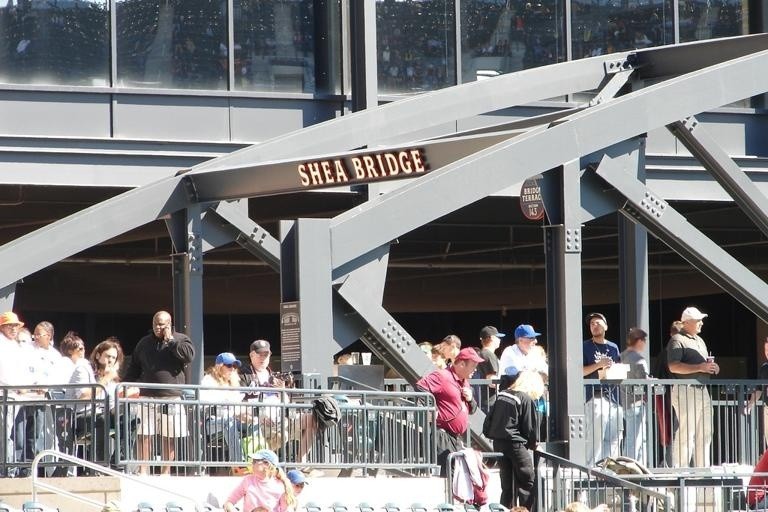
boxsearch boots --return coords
[468,397,478,414]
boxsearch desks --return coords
[245,56,315,95]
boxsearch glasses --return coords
[525,337,537,341]
[31,333,48,339]
[224,363,239,370]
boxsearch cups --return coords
[362,352,371,365]
[706,356,714,363]
[350,351,359,364]
[598,369,606,380]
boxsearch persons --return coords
[1,308,324,475]
[334,322,550,475]
[582,307,768,474]
[416,348,490,508]
[484,364,544,512]
[224,449,299,512]
[285,468,309,496]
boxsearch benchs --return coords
[49,393,225,473]
[289,395,381,456]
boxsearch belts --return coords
[689,383,706,390]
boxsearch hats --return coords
[0,312,25,328]
[249,339,271,353]
[515,324,542,339]
[681,307,709,325]
[248,449,279,468]
[588,313,608,327]
[287,471,310,487]
[456,347,485,363]
[479,326,506,340]
[215,352,242,368]
